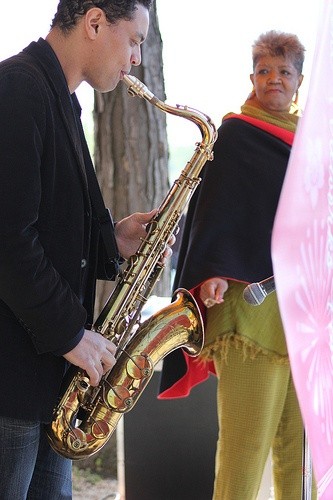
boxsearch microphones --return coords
[243,275,274,307]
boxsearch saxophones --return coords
[44,74,219,461]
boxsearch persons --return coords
[157,31,318,500]
[0,0,178,500]
